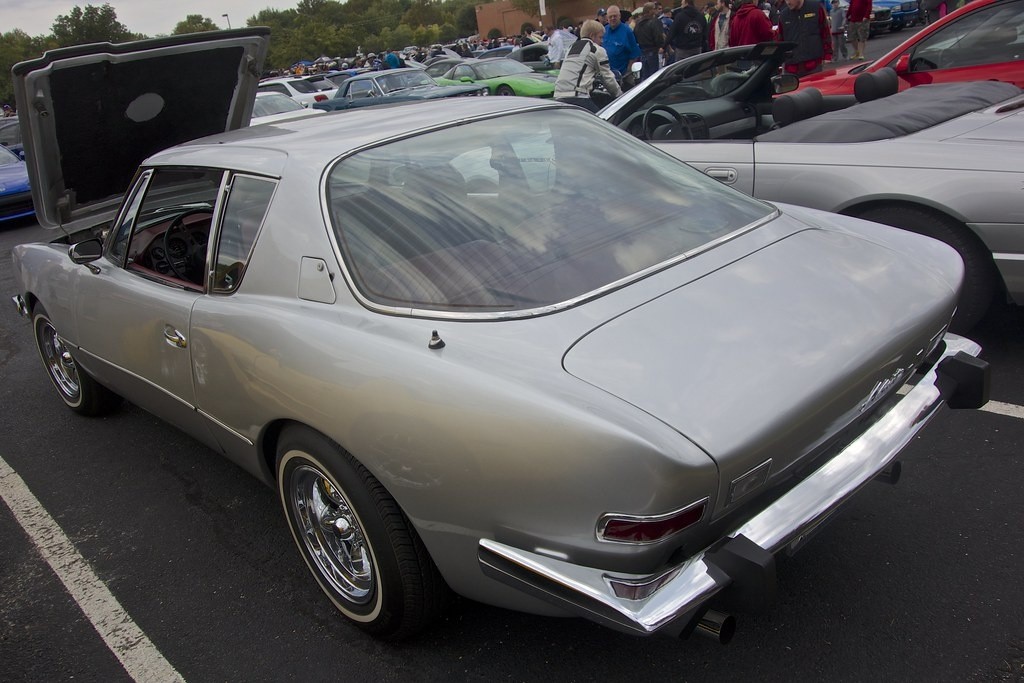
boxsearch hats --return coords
[705,3,715,12]
[598,8,607,15]
[367,53,376,59]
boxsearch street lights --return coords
[223,14,232,29]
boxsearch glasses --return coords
[657,7,663,11]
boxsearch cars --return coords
[594,42,1024,351]
[419,57,465,78]
[433,58,554,98]
[0,116,23,148]
[472,46,517,61]
[11,26,991,646]
[313,68,493,113]
[326,69,375,86]
[246,92,327,127]
[0,144,40,230]
[771,0,1024,96]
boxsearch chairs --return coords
[853,67,898,103]
[773,87,825,128]
[370,159,495,274]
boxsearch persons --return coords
[829,0,847,60]
[3,106,15,117]
[847,0,873,60]
[926,0,948,28]
[257,0,832,112]
[601,5,639,91]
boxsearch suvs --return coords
[827,0,894,39]
[295,73,341,100]
[258,76,329,109]
[871,0,918,32]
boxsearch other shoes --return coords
[849,53,858,59]
[858,53,864,60]
